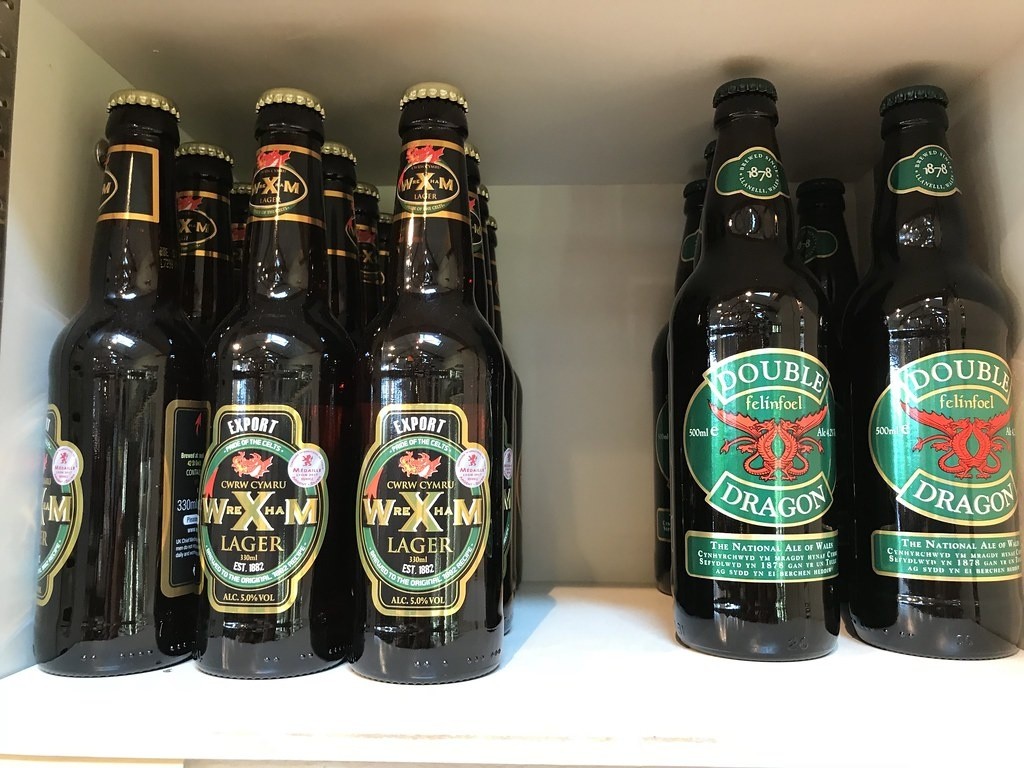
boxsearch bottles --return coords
[34,81,523,686]
[651,77,1024,661]
